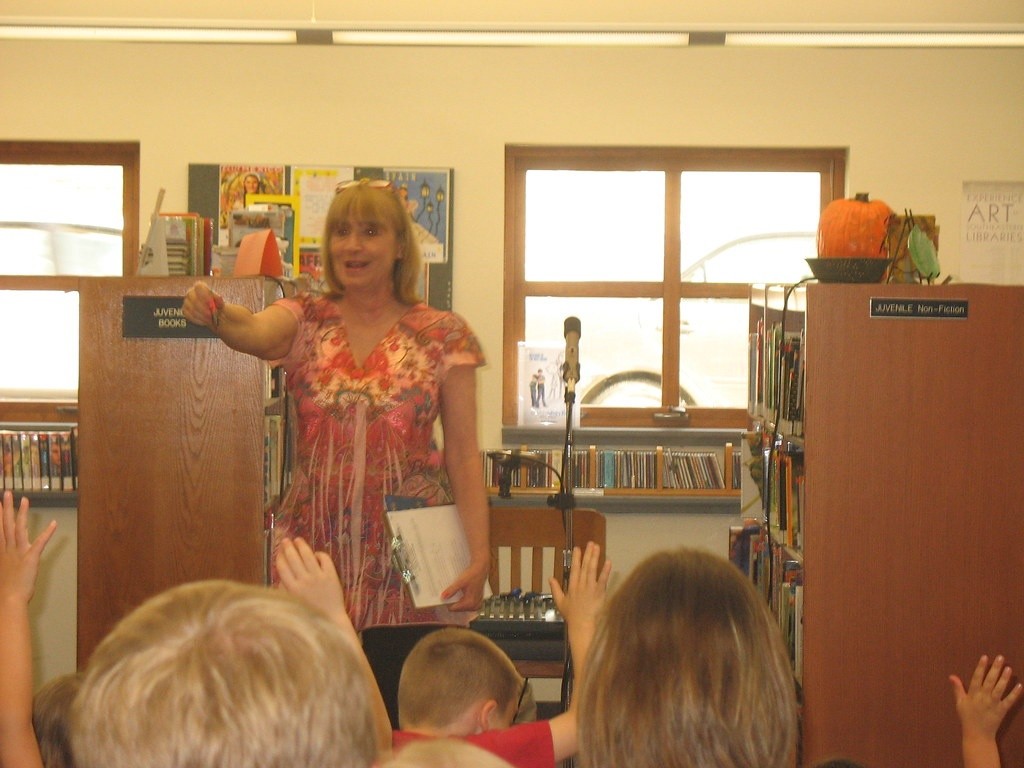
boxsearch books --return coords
[727,431,805,686]
[150,204,298,281]
[261,359,294,590]
[0,427,78,493]
[481,449,742,489]
[745,318,805,437]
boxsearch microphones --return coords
[564,317,580,401]
[488,452,545,469]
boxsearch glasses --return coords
[336,179,398,198]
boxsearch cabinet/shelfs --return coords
[742,285,1024,768]
[78,277,296,674]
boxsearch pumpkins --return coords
[817,192,897,279]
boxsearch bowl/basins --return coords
[805,256,892,283]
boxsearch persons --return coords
[179,186,494,731]
[0,489,1024,768]
